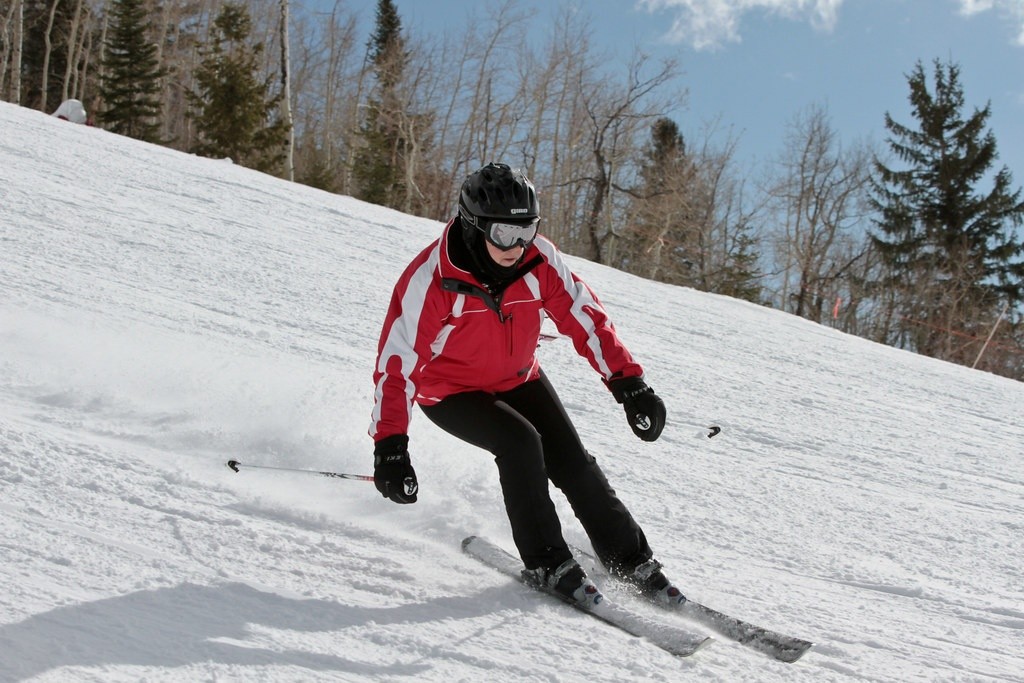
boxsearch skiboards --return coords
[459,531,818,666]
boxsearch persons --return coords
[368,161,688,610]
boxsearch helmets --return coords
[458,162,541,249]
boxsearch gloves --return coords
[612,378,666,442]
[373,432,419,504]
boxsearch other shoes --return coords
[539,556,596,602]
[628,556,670,592]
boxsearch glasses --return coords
[474,217,541,252]
